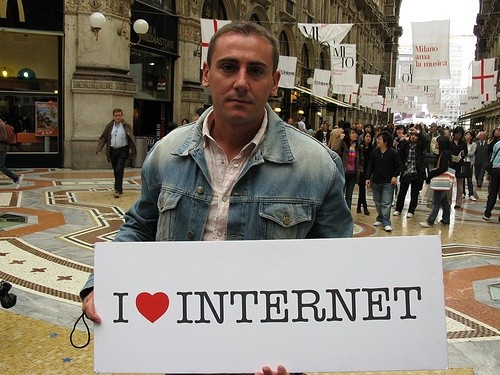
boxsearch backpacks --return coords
[5,121,17,144]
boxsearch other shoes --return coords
[113,192,120,198]
[482,215,489,220]
[454,206,460,208]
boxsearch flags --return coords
[297,23,353,47]
[471,58,496,93]
[460,94,473,112]
[201,18,232,69]
[412,20,451,79]
[480,71,499,102]
[312,44,442,115]
[276,55,298,89]
[466,88,482,107]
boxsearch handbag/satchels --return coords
[429,152,456,190]
[460,156,471,176]
[430,135,441,154]
[485,161,493,171]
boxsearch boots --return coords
[363,202,370,215]
[357,201,361,213]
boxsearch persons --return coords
[419,136,455,227]
[393,127,429,217]
[0,117,24,189]
[96,108,137,198]
[482,141,500,222]
[365,131,402,231]
[357,131,375,215]
[288,114,500,208]
[79,20,354,375]
[336,128,364,210]
[194,108,206,121]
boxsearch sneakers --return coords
[419,222,432,228]
[437,221,444,225]
[373,222,381,226]
[393,211,400,216]
[407,213,413,217]
[462,193,465,198]
[15,175,24,189]
[385,225,392,231]
[469,196,476,201]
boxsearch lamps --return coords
[307,77,313,85]
[117,18,149,45]
[89,12,105,41]
